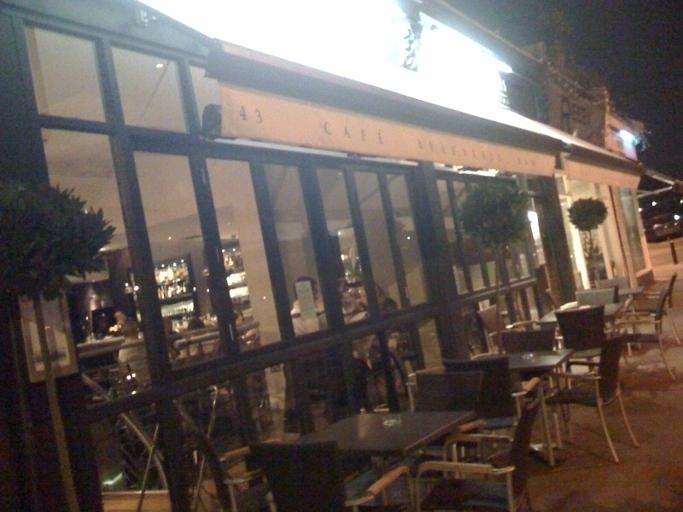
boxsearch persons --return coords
[358,279,407,401]
[290,276,366,419]
[162,307,257,359]
[118,318,154,386]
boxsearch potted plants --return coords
[568,197,615,306]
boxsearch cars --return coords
[645,213,682,238]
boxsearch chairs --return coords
[414,396,539,512]
[545,329,639,463]
[636,271,682,347]
[553,306,606,372]
[478,304,541,352]
[192,429,277,512]
[500,328,557,355]
[611,288,671,383]
[599,277,632,288]
[391,345,445,408]
[636,270,655,287]
[574,287,617,305]
[247,440,416,512]
[444,357,555,467]
[413,372,482,458]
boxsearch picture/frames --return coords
[16,290,80,383]
